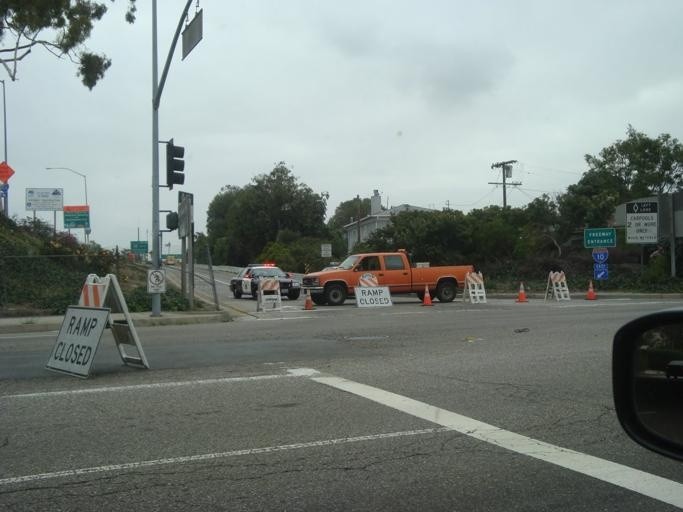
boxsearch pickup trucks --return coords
[302,250,476,307]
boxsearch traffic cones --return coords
[514,281,529,303]
[301,289,317,310]
[418,283,435,307]
[584,279,597,301]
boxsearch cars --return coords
[228,262,301,301]
[663,359,682,379]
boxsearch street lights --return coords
[45,166,90,245]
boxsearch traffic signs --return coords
[580,227,617,248]
[129,241,148,255]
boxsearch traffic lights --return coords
[157,139,186,190]
